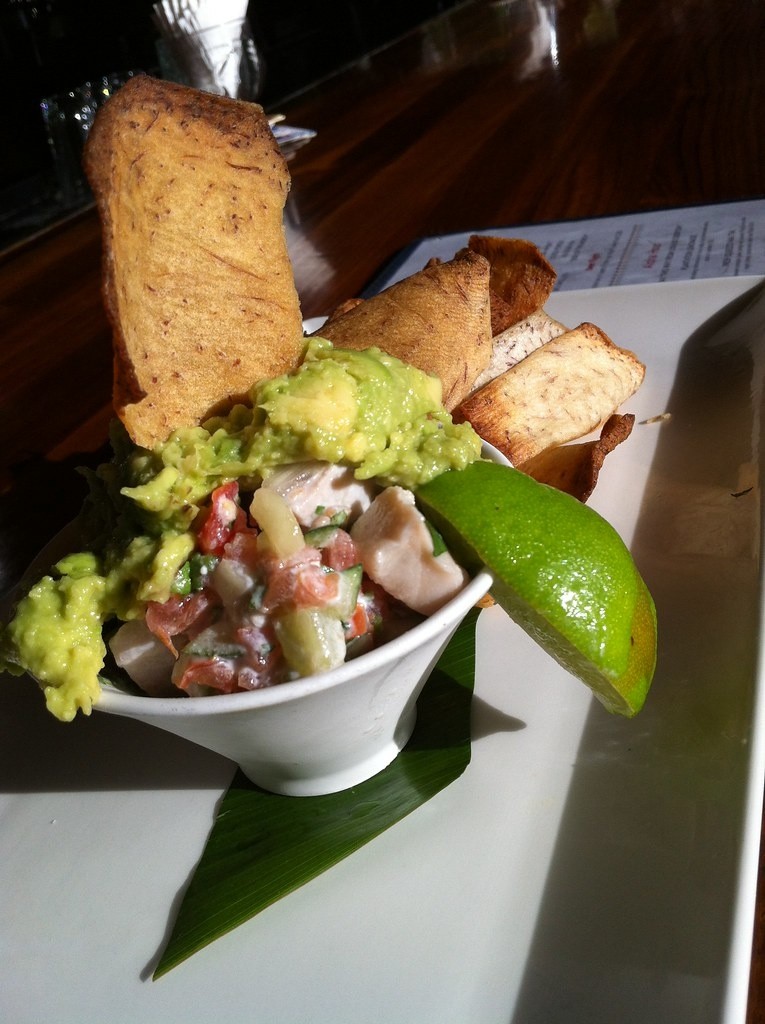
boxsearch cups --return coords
[165,17,260,103]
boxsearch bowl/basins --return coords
[92,437,515,797]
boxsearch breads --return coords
[83,77,643,496]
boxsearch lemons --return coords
[408,461,659,718]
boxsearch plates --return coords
[0,276,765,1024]
